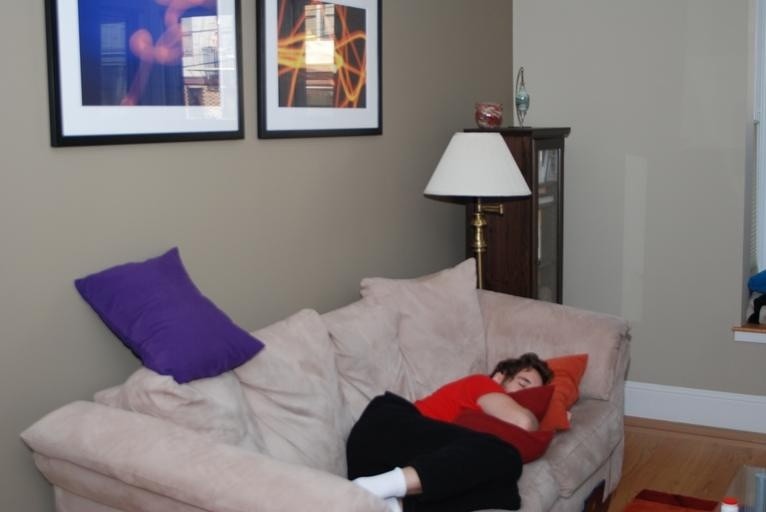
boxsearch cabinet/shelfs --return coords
[463,126,570,305]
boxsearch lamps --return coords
[422,132,533,290]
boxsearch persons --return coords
[344,349,555,511]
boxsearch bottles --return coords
[719,497,740,512]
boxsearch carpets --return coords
[625,488,717,511]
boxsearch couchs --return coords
[17,291,632,511]
